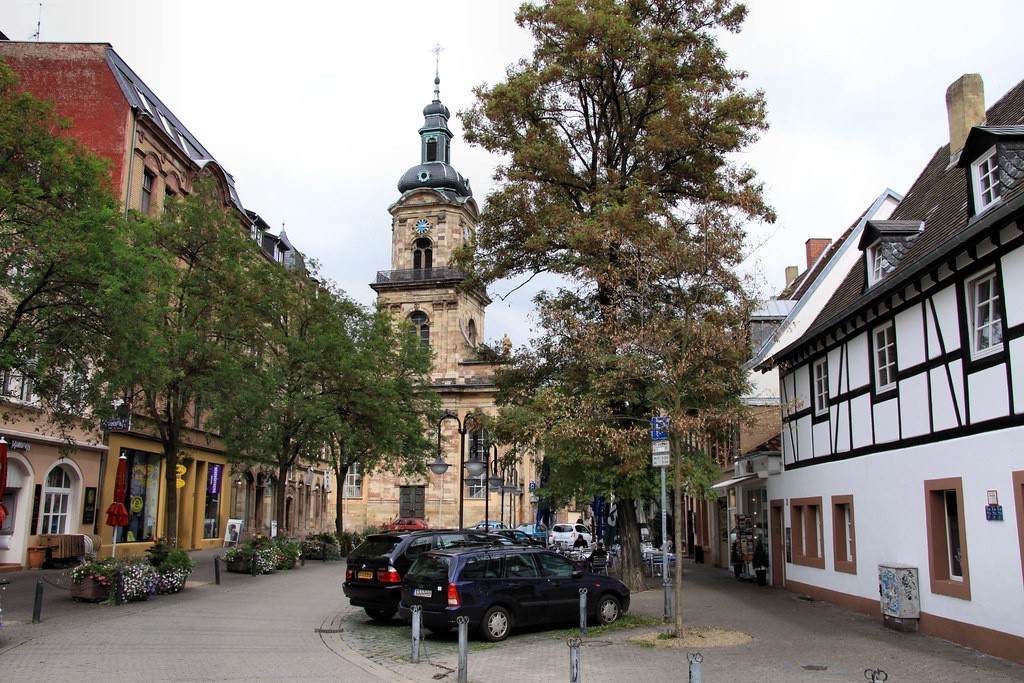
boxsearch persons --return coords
[582,543,606,573]
[573,534,588,551]
[659,535,673,552]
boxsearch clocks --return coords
[415,217,430,233]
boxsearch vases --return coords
[28,546,48,570]
[309,549,324,559]
[341,546,349,557]
[226,558,252,573]
[289,558,296,568]
[82,576,109,599]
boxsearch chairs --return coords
[552,542,685,579]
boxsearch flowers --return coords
[71,560,159,600]
[225,544,277,576]
[300,532,365,559]
[274,530,301,569]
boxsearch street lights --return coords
[463,443,524,531]
[427,414,487,531]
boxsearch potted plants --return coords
[145,538,191,592]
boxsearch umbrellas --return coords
[0,436,9,532]
[106,453,128,559]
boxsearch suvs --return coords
[342,529,517,623]
[397,547,632,642]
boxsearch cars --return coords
[548,523,598,551]
[474,520,510,530]
[516,523,551,545]
[379,517,428,529]
[486,529,551,548]
